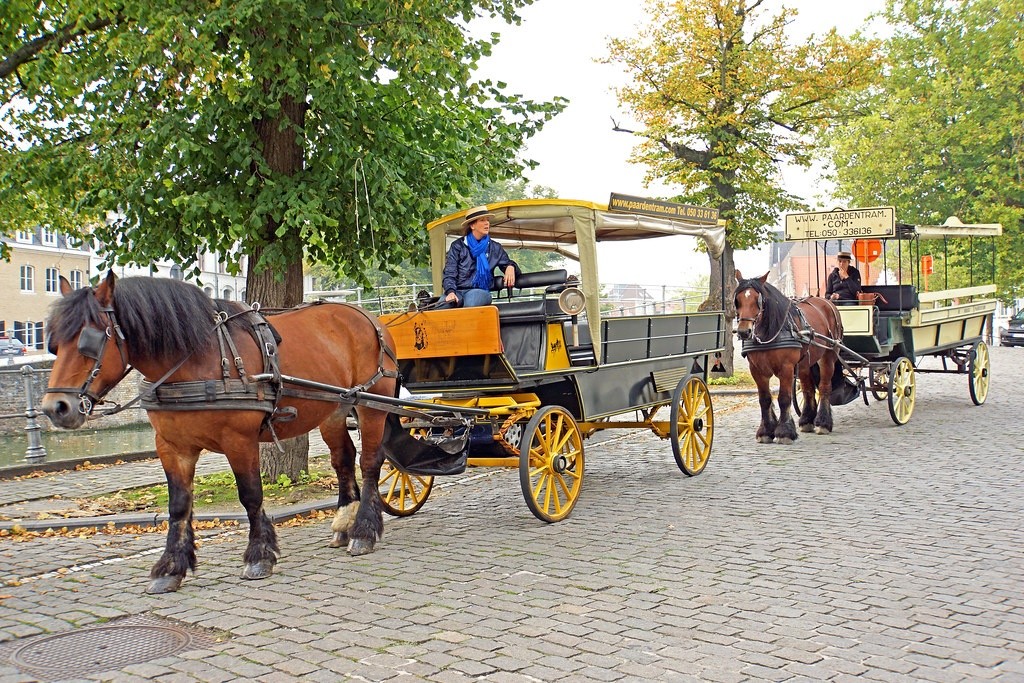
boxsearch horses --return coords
[41,270,397,595]
[731,267,841,446]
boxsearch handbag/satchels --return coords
[856,290,888,306]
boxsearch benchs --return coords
[488,268,571,324]
[858,283,917,319]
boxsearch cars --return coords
[0,337,27,359]
[1000,308,1024,347]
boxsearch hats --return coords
[837,252,851,260]
[461,205,496,226]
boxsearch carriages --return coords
[42,190,738,598]
[730,206,1001,450]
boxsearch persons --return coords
[433,206,523,310]
[711,352,726,372]
[824,251,861,305]
